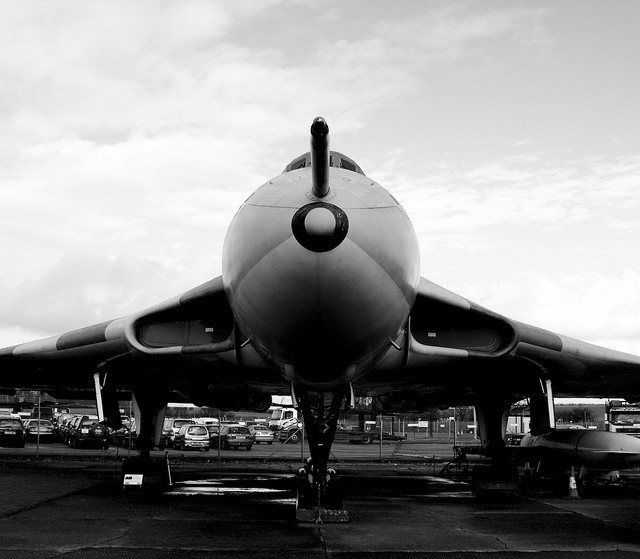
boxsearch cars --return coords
[248,425,274,444]
[211,425,253,450]
[23,419,57,444]
[172,425,210,451]
[0,418,26,447]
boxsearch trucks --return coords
[605,400,640,438]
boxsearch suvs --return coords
[162,420,198,448]
[62,416,109,450]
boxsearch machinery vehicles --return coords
[268,408,407,444]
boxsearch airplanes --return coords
[0,117,640,507]
[453,380,640,498]
